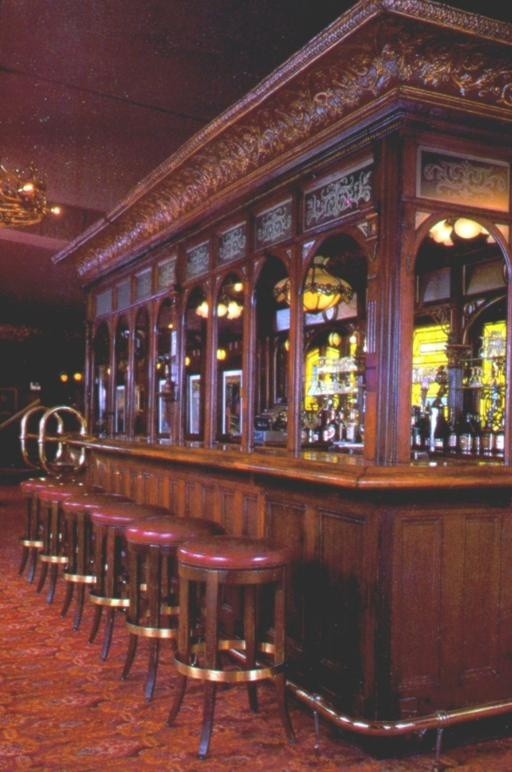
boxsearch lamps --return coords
[273,256,353,315]
[0,164,60,228]
[196,293,243,320]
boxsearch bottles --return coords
[411,406,505,454]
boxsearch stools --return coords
[89,503,173,661]
[37,484,101,604]
[166,533,297,760]
[118,515,227,704]
[21,477,77,581]
[61,495,135,631]
